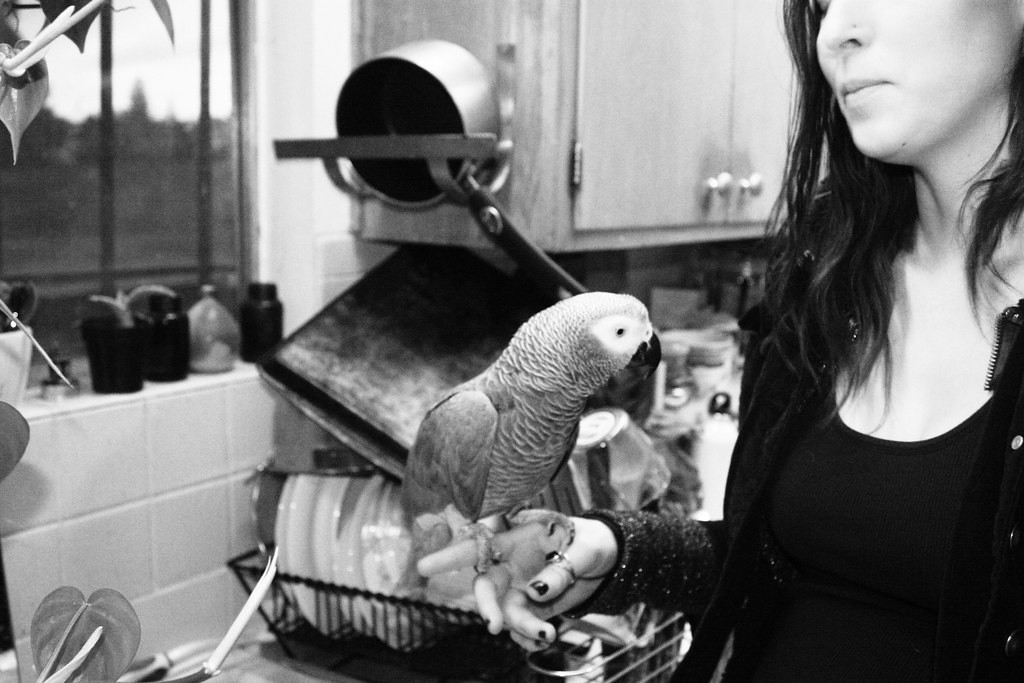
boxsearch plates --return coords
[250,450,425,660]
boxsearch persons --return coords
[418,0,1024,683]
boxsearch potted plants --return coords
[0,0,176,409]
[77,284,179,393]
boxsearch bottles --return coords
[239,282,282,363]
[141,282,189,381]
[185,284,239,372]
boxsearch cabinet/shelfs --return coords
[363,0,830,248]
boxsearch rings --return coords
[545,551,576,583]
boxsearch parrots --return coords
[401,292,662,588]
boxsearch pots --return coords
[332,39,590,297]
